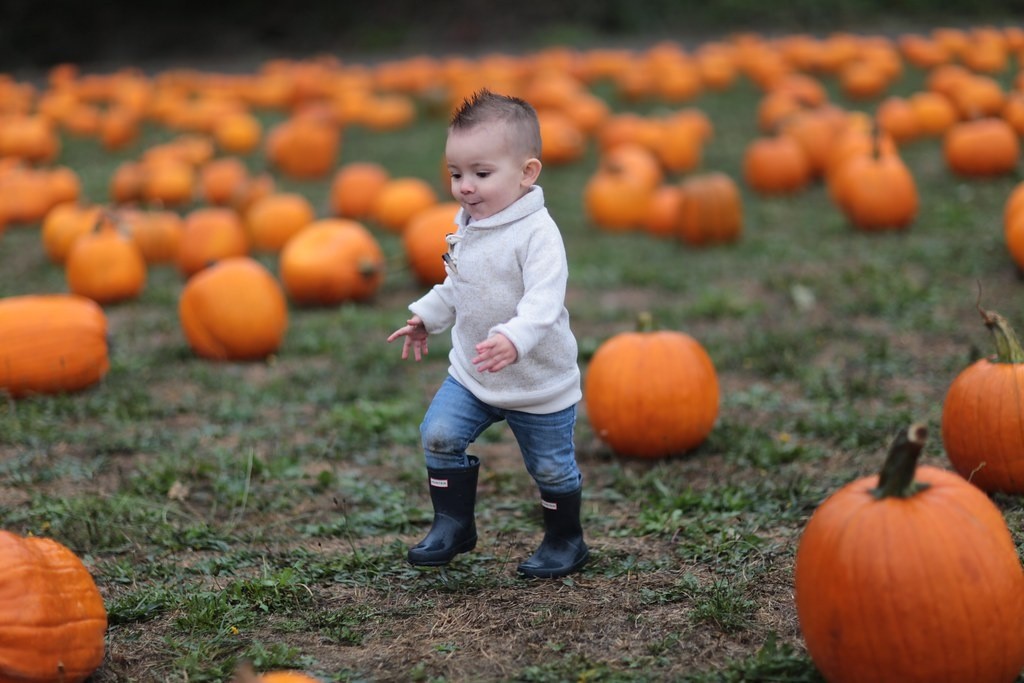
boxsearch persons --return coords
[388,88,591,577]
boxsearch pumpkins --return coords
[1,27,1024,495]
[0,532,108,683]
[796,421,1024,683]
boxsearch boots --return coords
[516,473,590,581]
[406,454,481,565]
